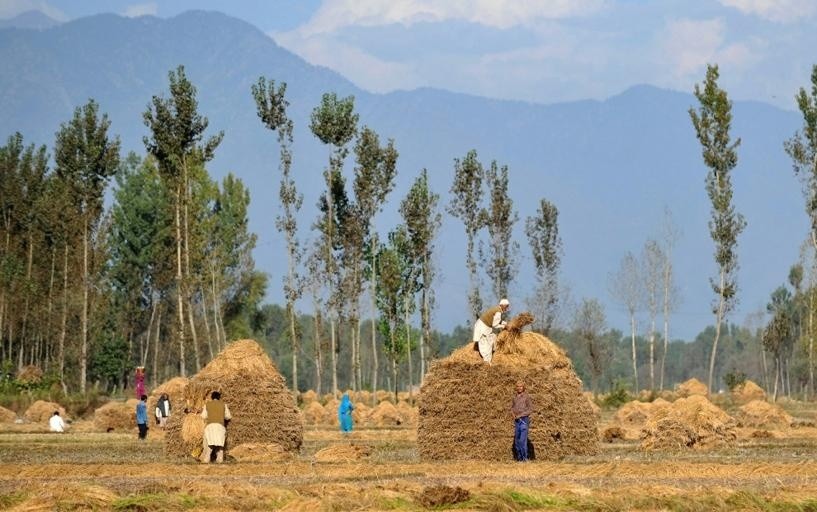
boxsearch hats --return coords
[500,299,510,305]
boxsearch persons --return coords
[508,381,528,462]
[135,394,148,440]
[198,392,232,462]
[154,392,172,426]
[48,411,64,432]
[471,297,510,364]
